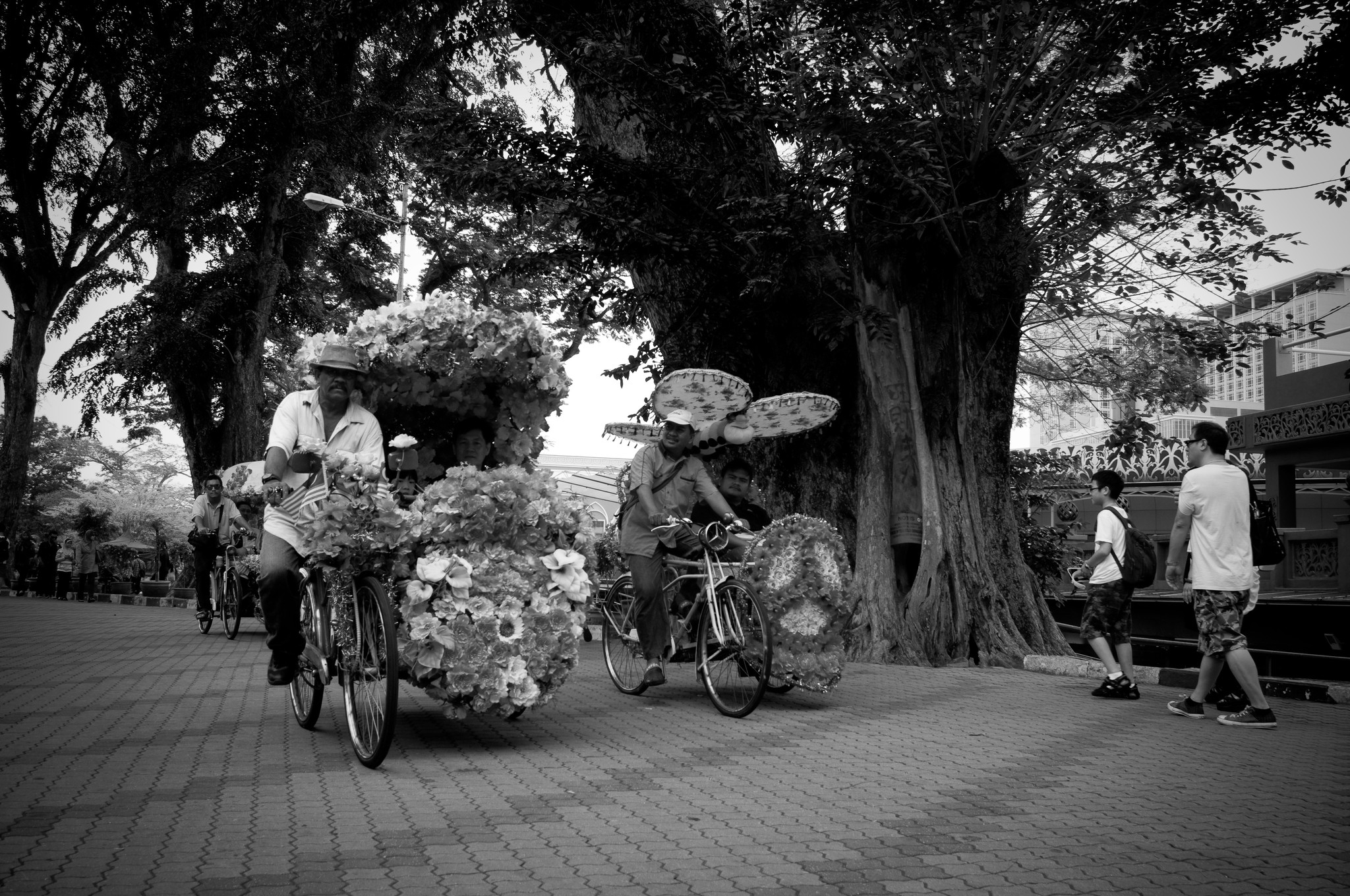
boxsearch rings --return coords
[275,486,282,493]
[267,487,275,495]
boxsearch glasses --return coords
[1183,439,1202,447]
[91,534,95,536]
[205,484,222,490]
[50,536,57,539]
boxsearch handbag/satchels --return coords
[1245,474,1286,566]
[187,505,224,550]
[96,550,106,563]
[139,569,145,577]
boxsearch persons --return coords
[614,409,750,686]
[384,464,418,496]
[0,526,179,602]
[257,339,390,688]
[1070,470,1146,701]
[450,417,493,471]
[1165,421,1280,728]
[688,459,771,532]
[191,475,257,620]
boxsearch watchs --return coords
[1084,561,1094,570]
[261,472,281,484]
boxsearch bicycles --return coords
[194,527,251,641]
[595,514,771,718]
[253,443,401,771]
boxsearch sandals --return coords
[196,609,209,620]
[1215,689,1251,712]
[1205,686,1231,703]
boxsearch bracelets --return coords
[1165,560,1179,567]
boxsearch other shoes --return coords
[12,587,141,603]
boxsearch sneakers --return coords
[1117,683,1140,699]
[670,595,698,626]
[1167,694,1205,719]
[1091,671,1131,696]
[644,662,665,685]
[1217,705,1278,728]
[267,656,299,685]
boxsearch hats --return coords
[135,554,141,558]
[658,409,696,434]
[309,345,367,374]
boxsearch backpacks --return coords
[0,536,9,563]
[1094,506,1157,588]
